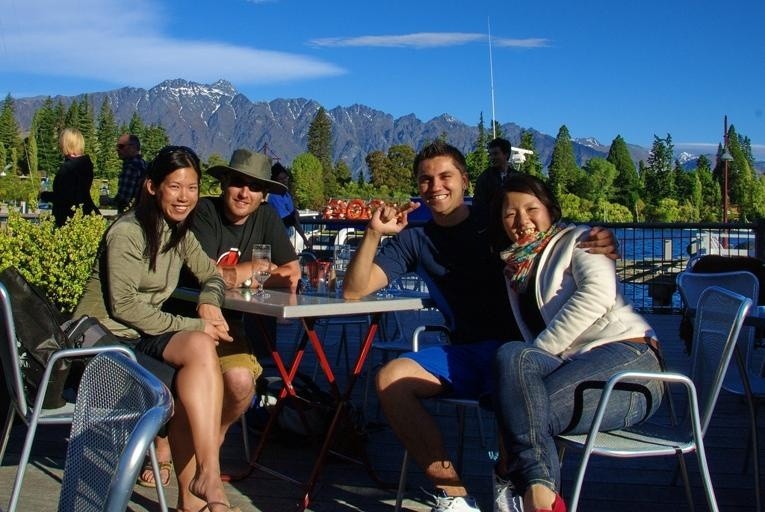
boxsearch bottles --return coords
[317,265,327,296]
[328,264,337,299]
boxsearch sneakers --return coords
[420,495,480,512]
[491,470,524,512]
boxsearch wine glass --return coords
[252,244,272,298]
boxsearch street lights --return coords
[721,114,736,253]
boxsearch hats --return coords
[206,149,289,201]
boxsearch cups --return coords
[305,259,334,288]
[333,243,351,268]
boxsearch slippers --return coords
[137,460,173,488]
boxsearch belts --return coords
[622,336,663,350]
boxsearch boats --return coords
[680,228,760,260]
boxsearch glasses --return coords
[116,143,133,148]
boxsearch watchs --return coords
[243,278,252,288]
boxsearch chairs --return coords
[0,281,167,512]
[57,348,177,512]
[361,298,439,420]
[312,285,384,382]
[395,325,504,512]
[675,272,765,448]
[555,285,755,512]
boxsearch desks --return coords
[172,286,425,512]
[685,305,764,328]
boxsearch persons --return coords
[266,162,312,249]
[99,134,147,215]
[138,149,302,487]
[486,174,665,512]
[472,138,519,206]
[71,146,242,512]
[39,127,102,228]
[342,141,621,512]
